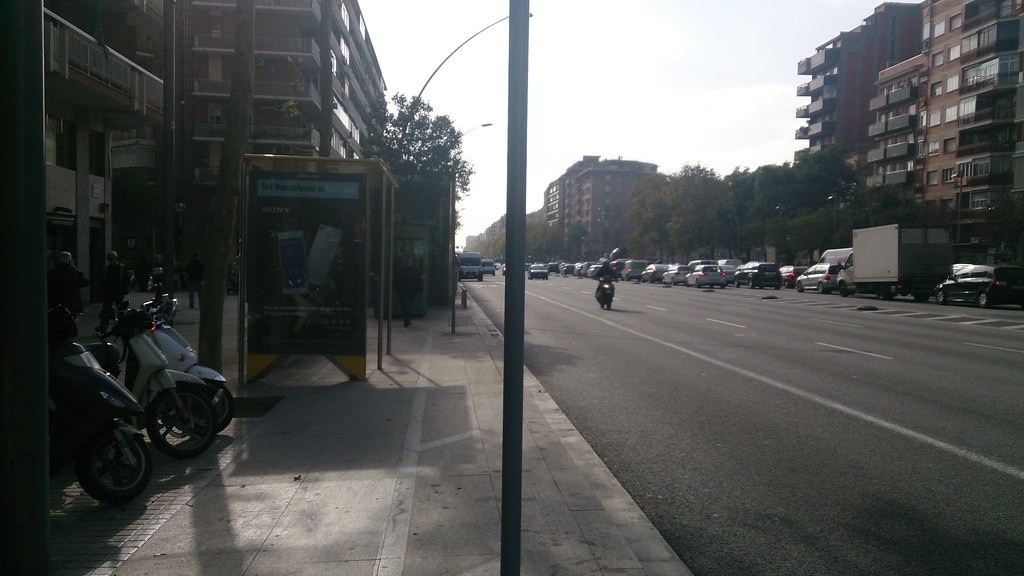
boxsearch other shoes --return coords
[95,326,105,334]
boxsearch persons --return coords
[186,252,205,308]
[595,260,618,298]
[396,255,419,327]
[94,251,130,334]
[46,251,83,320]
[148,255,174,302]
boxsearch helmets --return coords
[602,259,610,265]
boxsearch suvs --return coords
[621,260,647,281]
[779,265,809,289]
[735,261,783,289]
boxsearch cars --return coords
[685,265,727,289]
[528,264,549,280]
[719,264,738,285]
[662,265,692,286]
[500,259,531,275]
[795,262,842,294]
[641,264,666,284]
[547,257,627,281]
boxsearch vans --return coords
[932,264,1024,309]
[687,260,719,267]
[720,259,743,267]
[817,248,853,267]
[458,253,484,281]
[481,259,496,275]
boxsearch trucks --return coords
[836,224,953,301]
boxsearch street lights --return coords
[951,169,963,263]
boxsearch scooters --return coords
[595,274,618,310]
[76,264,235,458]
[48,304,154,506]
